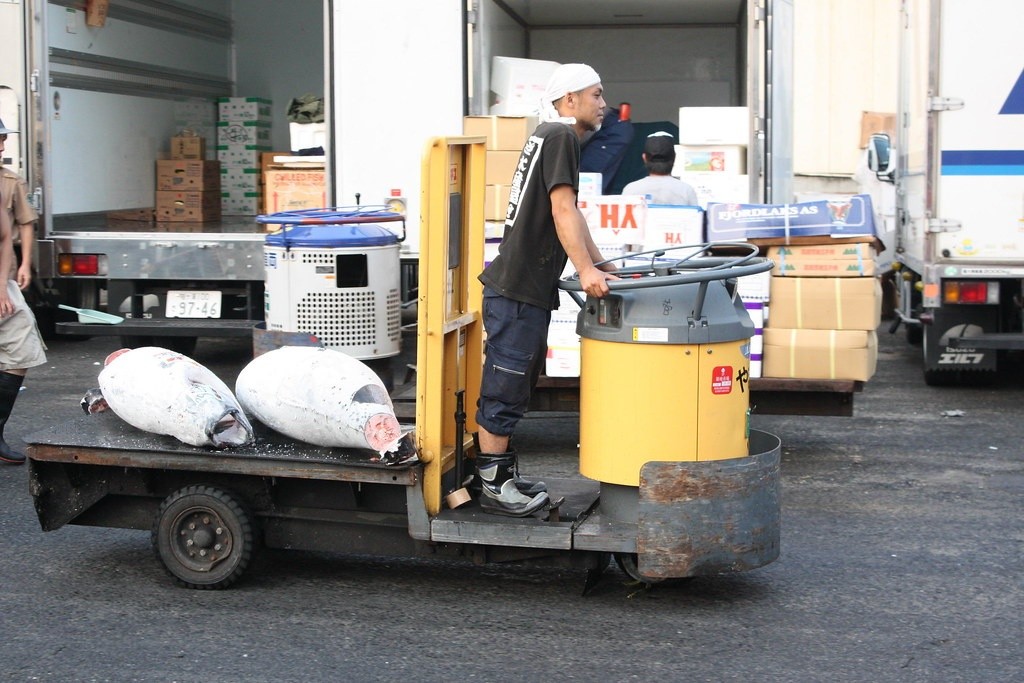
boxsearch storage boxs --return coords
[156,94,330,228]
[464,108,884,383]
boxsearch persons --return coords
[0,120,47,463]
[622,131,698,206]
[579,103,633,194]
[474,64,622,518]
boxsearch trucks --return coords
[868,0,1024,391]
[1,1,796,361]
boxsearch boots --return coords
[1,371,26,463]
[480,450,551,516]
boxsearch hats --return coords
[644,130,674,156]
[0,118,20,134]
